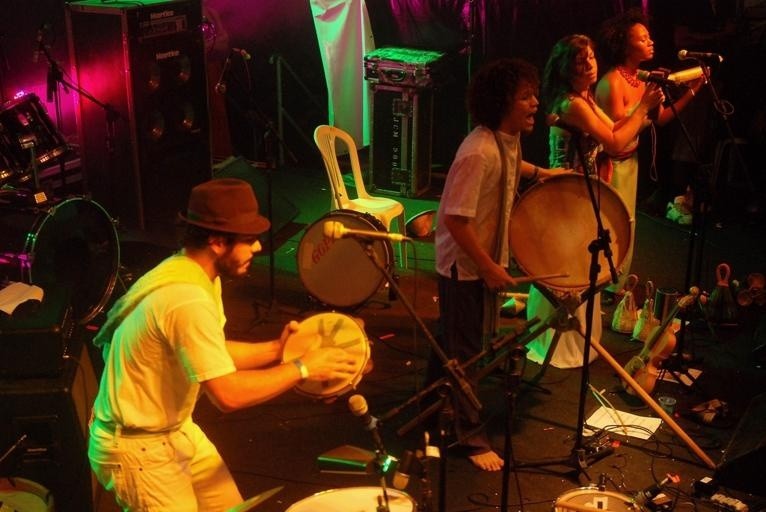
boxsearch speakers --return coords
[720,393,766,492]
[0,333,103,512]
[64,0,213,231]
[213,156,300,236]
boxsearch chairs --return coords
[312,122,409,272]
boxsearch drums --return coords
[281,313,372,400]
[283,482,420,512]
[1,92,71,177]
[0,188,121,325]
[553,488,644,512]
[507,173,634,292]
[295,209,395,313]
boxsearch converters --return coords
[695,476,718,496]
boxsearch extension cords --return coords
[700,493,749,512]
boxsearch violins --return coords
[620,287,700,397]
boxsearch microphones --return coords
[324,221,403,241]
[348,394,388,457]
[638,71,688,89]
[546,113,592,138]
[678,50,719,62]
[634,477,670,504]
[215,58,231,94]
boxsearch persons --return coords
[594,18,709,297]
[430,59,581,471]
[87,178,356,512]
[526,33,665,370]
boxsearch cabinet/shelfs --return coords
[0,346,98,469]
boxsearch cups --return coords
[658,396,677,417]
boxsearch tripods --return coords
[232,56,305,334]
[509,139,619,487]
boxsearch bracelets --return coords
[638,105,649,114]
[689,89,695,97]
[530,163,539,181]
[292,358,309,384]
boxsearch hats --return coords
[179,178,271,236]
